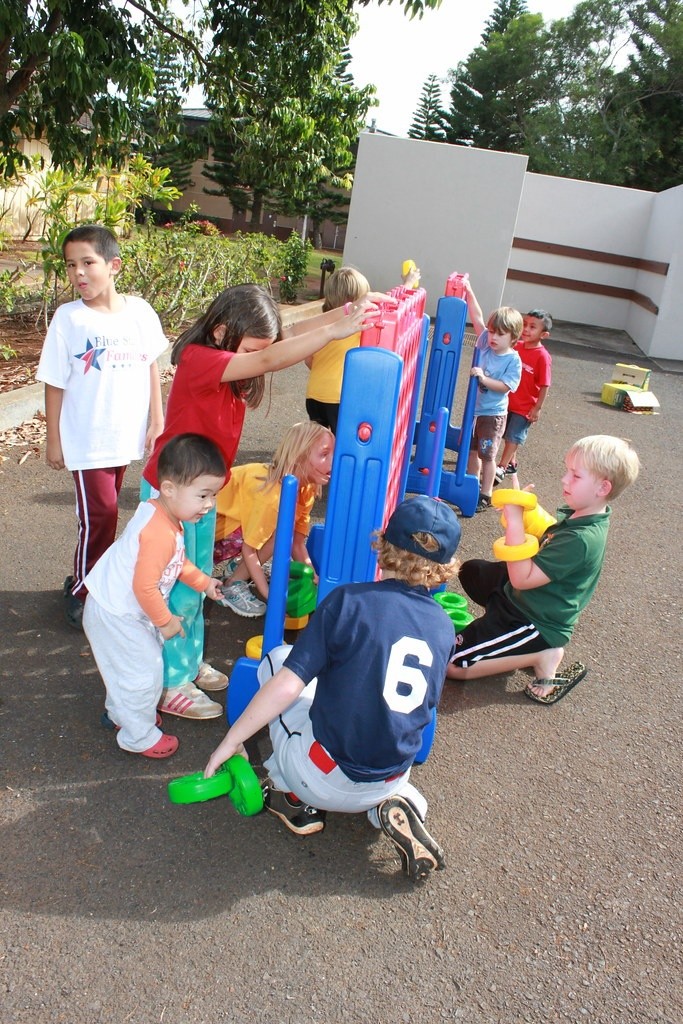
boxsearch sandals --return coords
[101,712,177,757]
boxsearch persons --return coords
[81,432,225,758]
[461,279,523,514]
[138,282,397,719]
[203,496,462,882]
[304,266,421,440]
[444,434,639,705]
[212,421,336,619]
[494,310,553,488]
[35,226,168,628]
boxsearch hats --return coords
[382,495,461,565]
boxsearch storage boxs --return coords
[610,362,652,391]
[601,383,661,416]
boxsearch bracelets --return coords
[344,301,352,316]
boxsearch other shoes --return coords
[494,466,506,483]
[505,463,518,474]
[62,576,84,629]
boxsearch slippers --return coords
[475,493,492,512]
[523,662,587,706]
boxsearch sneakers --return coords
[157,663,229,720]
[259,776,324,834]
[378,794,446,887]
[215,578,267,618]
